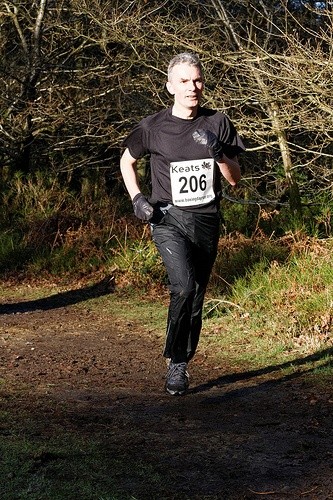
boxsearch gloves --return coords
[132,194,154,221]
[192,129,224,161]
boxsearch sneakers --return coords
[165,361,191,395]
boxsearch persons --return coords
[120,51,246,396]
